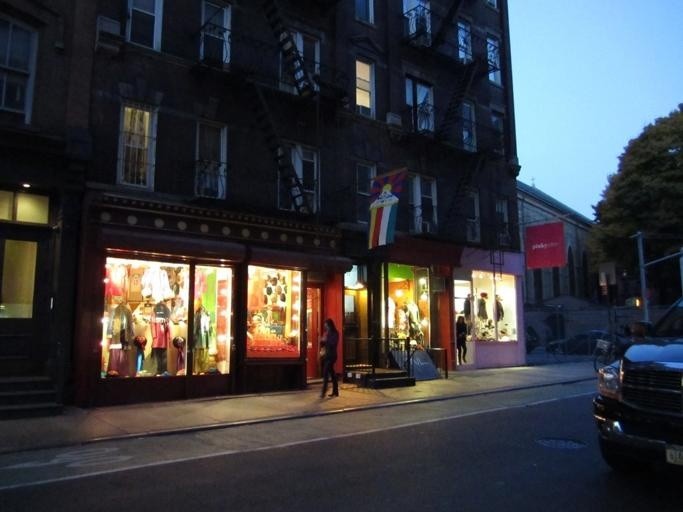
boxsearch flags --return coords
[365,171,404,251]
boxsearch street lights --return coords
[544,303,565,339]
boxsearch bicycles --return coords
[591,339,629,375]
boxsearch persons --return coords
[149,299,170,374]
[107,300,133,372]
[456,316,467,365]
[317,318,340,398]
[193,304,213,371]
[381,294,422,344]
[464,292,504,330]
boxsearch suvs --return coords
[589,290,682,492]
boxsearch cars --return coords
[545,327,628,357]
[524,301,565,312]
[526,325,541,352]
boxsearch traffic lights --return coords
[635,297,641,309]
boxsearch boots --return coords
[319,384,339,399]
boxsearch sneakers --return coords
[459,358,466,365]
